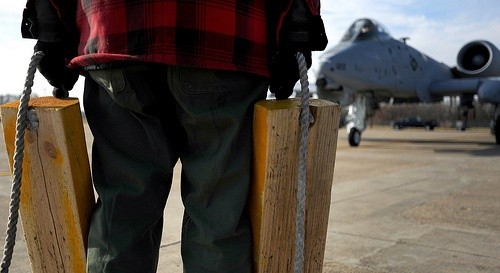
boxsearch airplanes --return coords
[267,18,500,146]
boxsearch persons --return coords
[21,0,328,273]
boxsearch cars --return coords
[454,122,466,131]
[390,113,440,132]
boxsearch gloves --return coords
[34,40,79,98]
[267,42,312,101]
